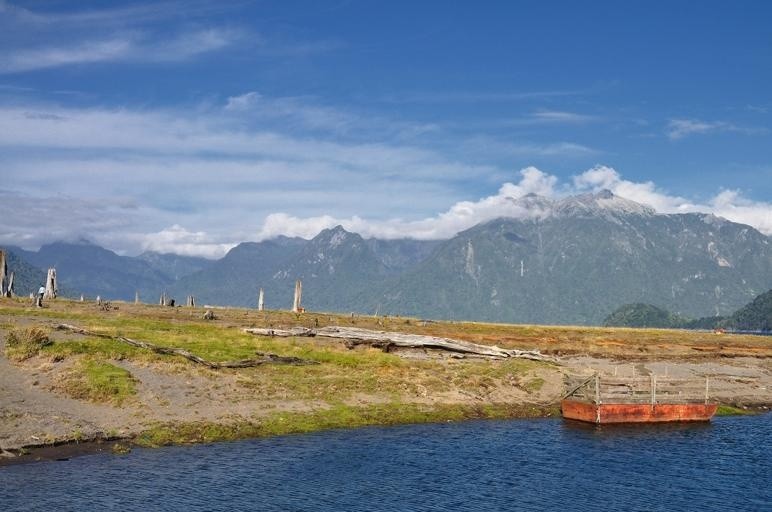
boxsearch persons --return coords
[37,284,46,298]
